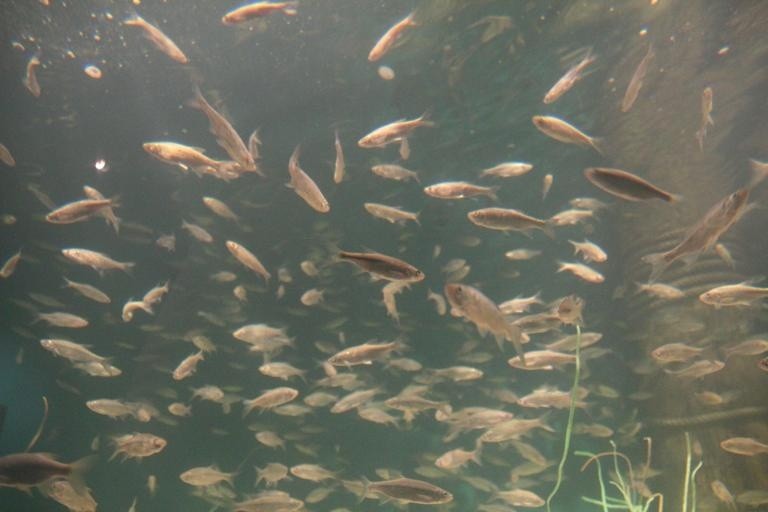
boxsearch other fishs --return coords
[0,0,768,512]
